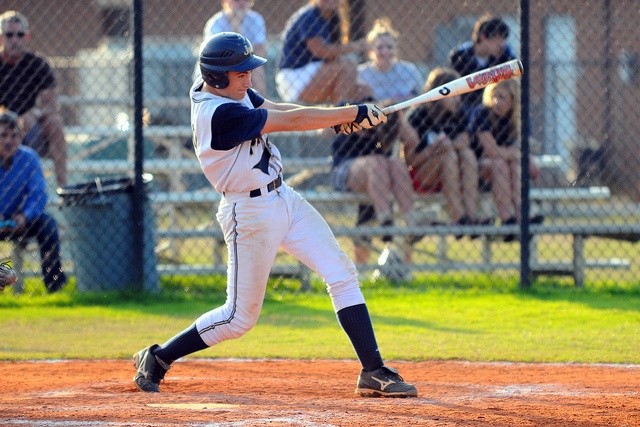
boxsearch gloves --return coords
[353,103,388,129]
[332,102,363,134]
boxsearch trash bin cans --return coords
[55,172,158,294]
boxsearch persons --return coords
[449,13,519,124]
[357,17,425,167]
[459,74,545,243]
[401,66,493,240]
[131,31,419,399]
[203,0,270,101]
[1,11,70,188]
[0,108,72,293]
[276,0,372,108]
[331,96,439,242]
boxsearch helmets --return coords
[199,31,268,89]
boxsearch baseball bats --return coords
[332,58,525,135]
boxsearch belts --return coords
[250,174,283,197]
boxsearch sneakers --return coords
[132,344,167,392]
[355,365,417,396]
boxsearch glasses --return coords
[4,31,26,38]
